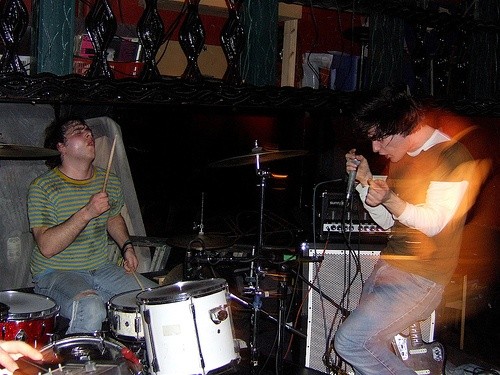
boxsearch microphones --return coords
[184,251,193,281]
[346,158,361,200]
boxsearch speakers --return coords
[301,241,435,375]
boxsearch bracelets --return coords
[124,246,134,254]
[121,240,133,254]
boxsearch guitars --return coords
[388,321,446,375]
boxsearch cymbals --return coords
[165,234,236,252]
[0,140,61,163]
[207,138,309,171]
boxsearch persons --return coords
[26,114,158,333]
[0,339,43,371]
[333,92,500,375]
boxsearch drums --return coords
[0,290,59,370]
[37,334,132,375]
[136,276,243,375]
[105,288,151,343]
